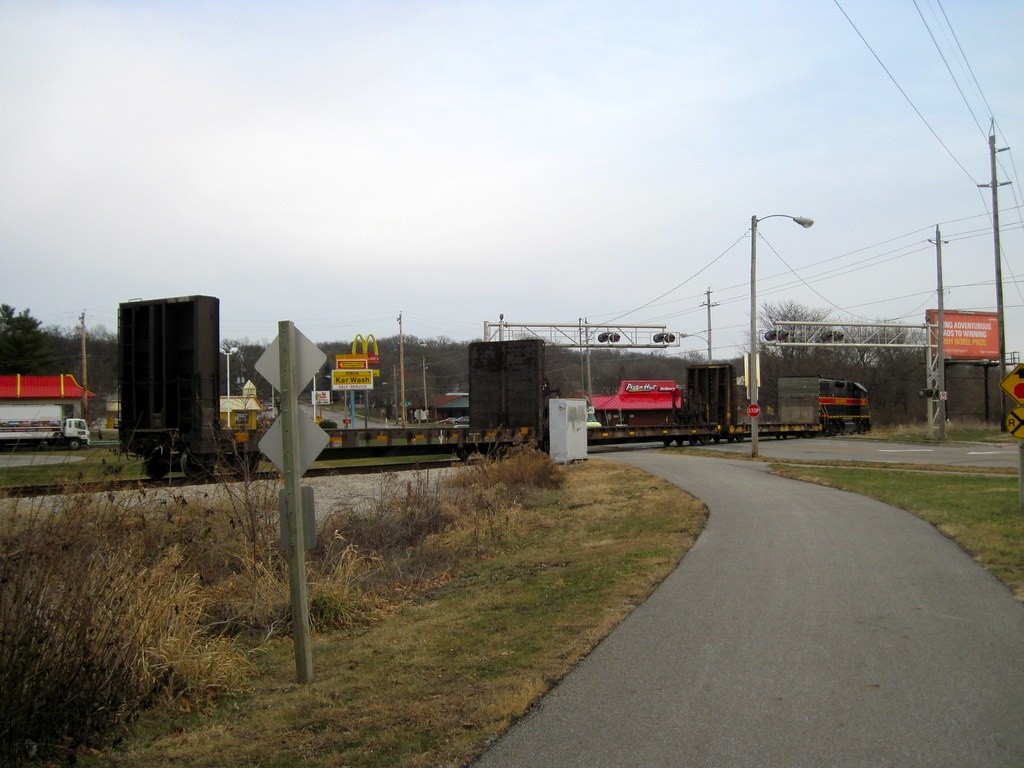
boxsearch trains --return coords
[111,292,874,489]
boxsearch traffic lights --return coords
[778,331,790,341]
[834,331,845,341]
[765,331,777,341]
[598,333,608,342]
[821,332,833,342]
[664,334,675,344]
[925,389,933,398]
[610,333,620,343]
[653,334,664,343]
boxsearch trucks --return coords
[0,404,92,451]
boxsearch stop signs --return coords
[747,403,761,417]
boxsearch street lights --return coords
[219,346,238,428]
[680,333,712,361]
[749,212,815,460]
